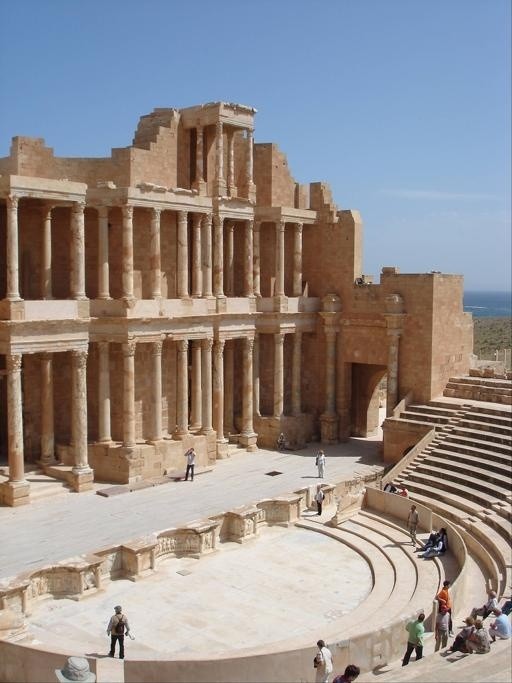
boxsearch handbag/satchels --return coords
[314,657,320,668]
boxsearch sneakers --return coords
[108,653,114,657]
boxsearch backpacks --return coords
[115,614,125,635]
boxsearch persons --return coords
[107,606,130,658]
[317,449,326,478]
[278,432,285,451]
[333,665,359,683]
[316,640,334,683]
[384,481,512,666]
[316,484,324,515]
[184,448,196,480]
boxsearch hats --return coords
[55,657,96,683]
[114,606,122,611]
